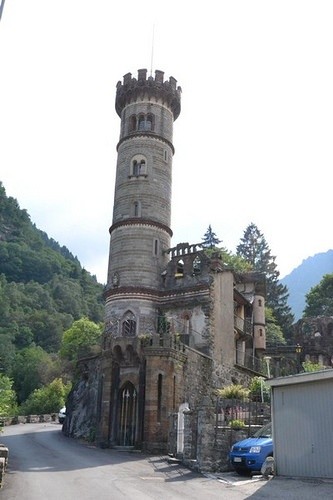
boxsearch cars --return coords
[228,422,272,476]
[58,405,67,424]
